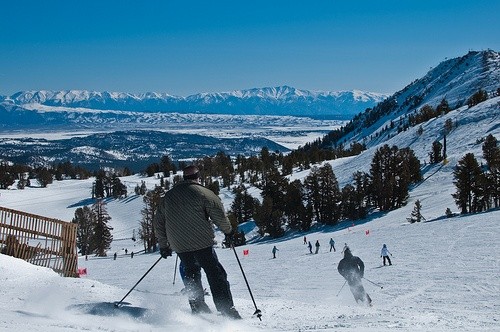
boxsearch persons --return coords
[272,245,279,258]
[155,166,244,321]
[303,235,307,244]
[113,253,117,260]
[131,251,134,258]
[380,244,392,266]
[315,240,320,254]
[338,249,372,304]
[341,243,350,254]
[329,238,336,252]
[308,241,313,253]
[125,249,128,254]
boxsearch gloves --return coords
[159,247,172,259]
[222,234,234,248]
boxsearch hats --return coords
[183,165,200,180]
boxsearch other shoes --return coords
[222,308,242,320]
